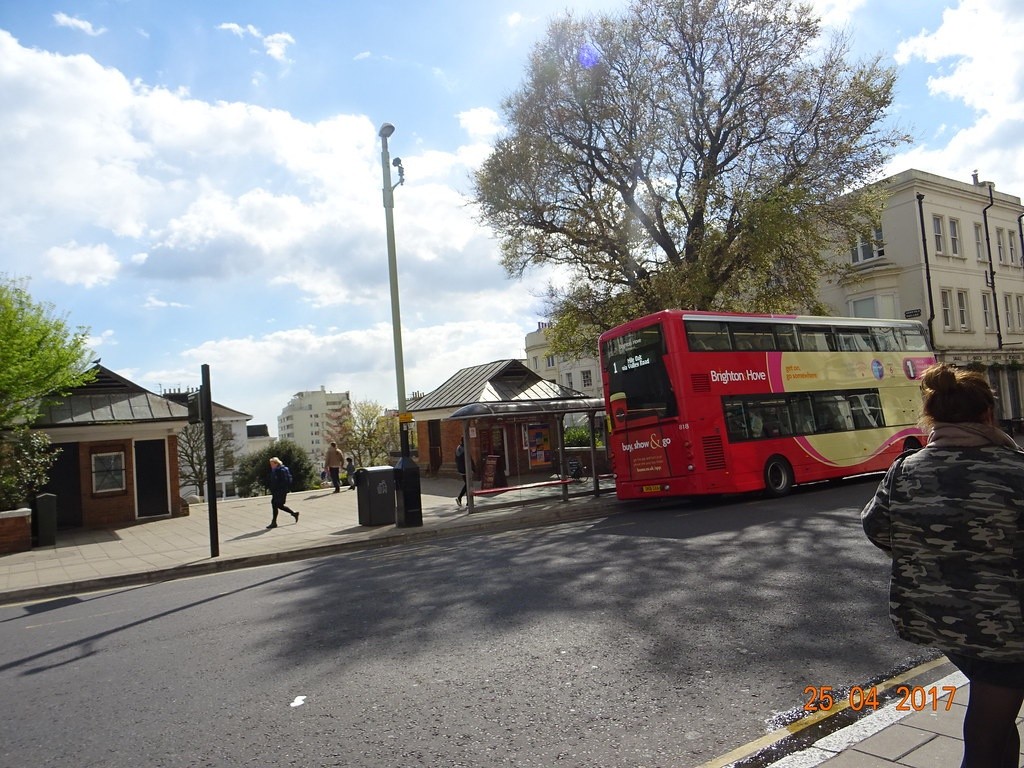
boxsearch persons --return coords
[455,437,477,507]
[861,365,1024,768]
[266,457,299,528]
[321,442,345,493]
[342,457,356,490]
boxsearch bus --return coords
[596,305,940,503]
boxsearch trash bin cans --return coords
[36,494,57,546]
[354,464,396,526]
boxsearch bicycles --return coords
[556,461,589,483]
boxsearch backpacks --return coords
[277,467,292,493]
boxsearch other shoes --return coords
[292,512,299,522]
[266,520,277,529]
[349,485,355,490]
[466,503,477,506]
[455,498,461,506]
[333,488,340,493]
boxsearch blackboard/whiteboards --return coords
[480,454,508,490]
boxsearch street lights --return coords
[377,122,422,526]
